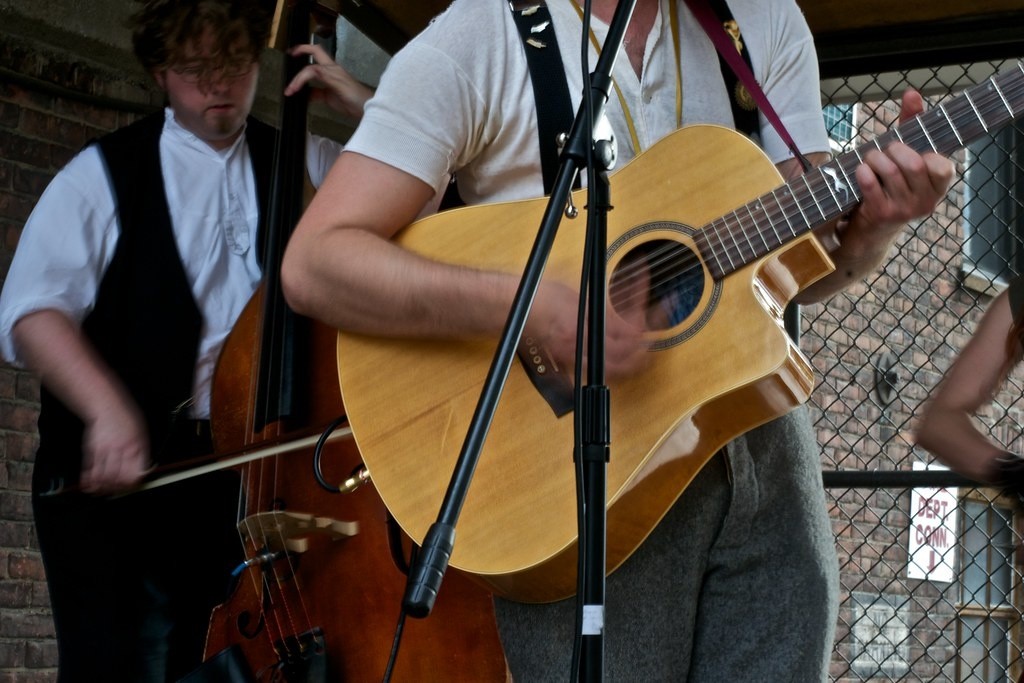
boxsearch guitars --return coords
[335,53,1024,604]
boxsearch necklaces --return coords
[571,0,682,155]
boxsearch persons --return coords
[281,0,955,683]
[0,1,375,683]
[914,271,1024,508]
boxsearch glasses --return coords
[171,58,258,84]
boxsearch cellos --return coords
[202,1,513,683]
